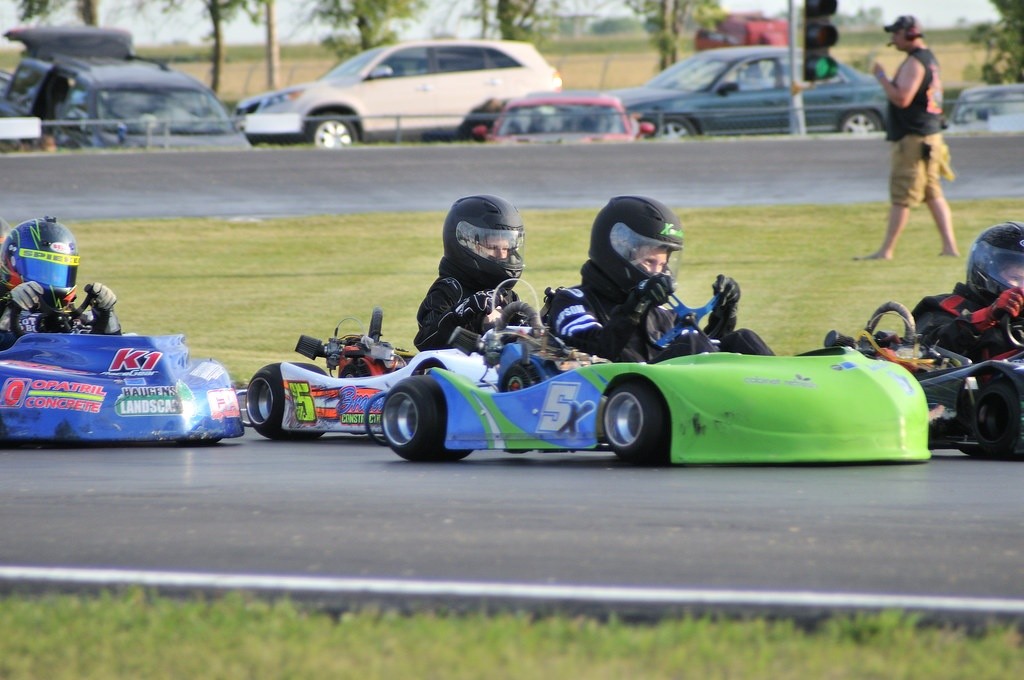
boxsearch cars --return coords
[694,10,791,52]
[470,93,656,143]
[940,83,1024,134]
[1,24,253,150]
[556,42,890,137]
[228,36,563,149]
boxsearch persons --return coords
[413,197,591,371]
[852,15,961,259]
[738,67,762,92]
[904,221,1024,363]
[0,216,122,351]
[548,196,777,364]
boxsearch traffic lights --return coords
[798,0,840,85]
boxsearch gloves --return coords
[453,291,503,322]
[85,283,118,312]
[643,274,674,305]
[971,288,1024,332]
[4,281,44,311]
[713,274,741,318]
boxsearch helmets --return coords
[966,221,1024,305]
[443,194,525,289]
[589,195,684,292]
[0,217,79,309]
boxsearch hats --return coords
[884,16,922,35]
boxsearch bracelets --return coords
[876,71,884,80]
